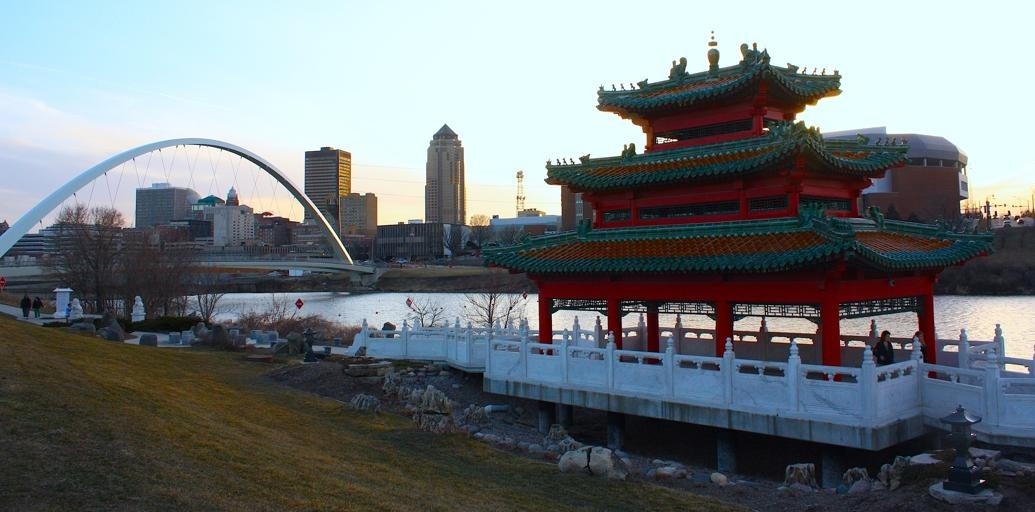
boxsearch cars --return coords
[396,258,408,264]
[1003,215,1024,226]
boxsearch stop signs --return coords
[295,298,303,310]
[523,293,527,299]
[0,276,6,289]
[406,298,412,307]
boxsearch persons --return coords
[874,328,895,382]
[32,296,44,320]
[912,329,930,364]
[19,293,30,320]
